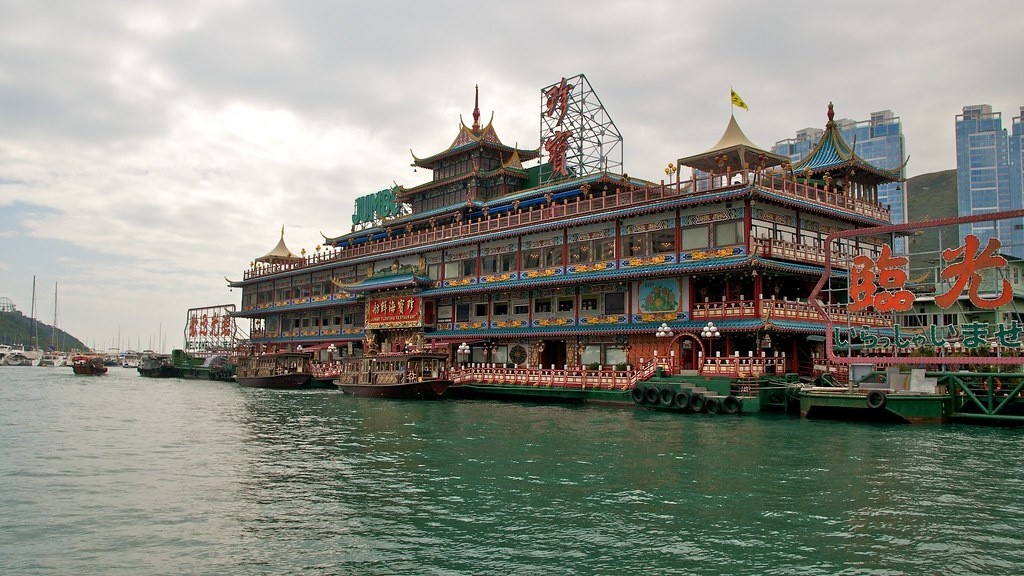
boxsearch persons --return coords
[80,362,82,364]
[163,360,168,366]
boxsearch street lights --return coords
[327,343,337,366]
[456,342,471,371]
[701,322,722,357]
[656,323,674,362]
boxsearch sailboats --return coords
[0,274,174,369]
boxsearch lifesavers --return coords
[984,377,1002,393]
[705,398,721,415]
[644,387,660,404]
[674,392,690,408]
[690,393,705,412]
[632,386,646,404]
[723,397,740,414]
[867,390,885,409]
[658,388,675,407]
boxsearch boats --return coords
[72,355,108,376]
[332,349,454,402]
[137,349,184,379]
[231,343,313,391]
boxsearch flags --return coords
[732,90,749,110]
